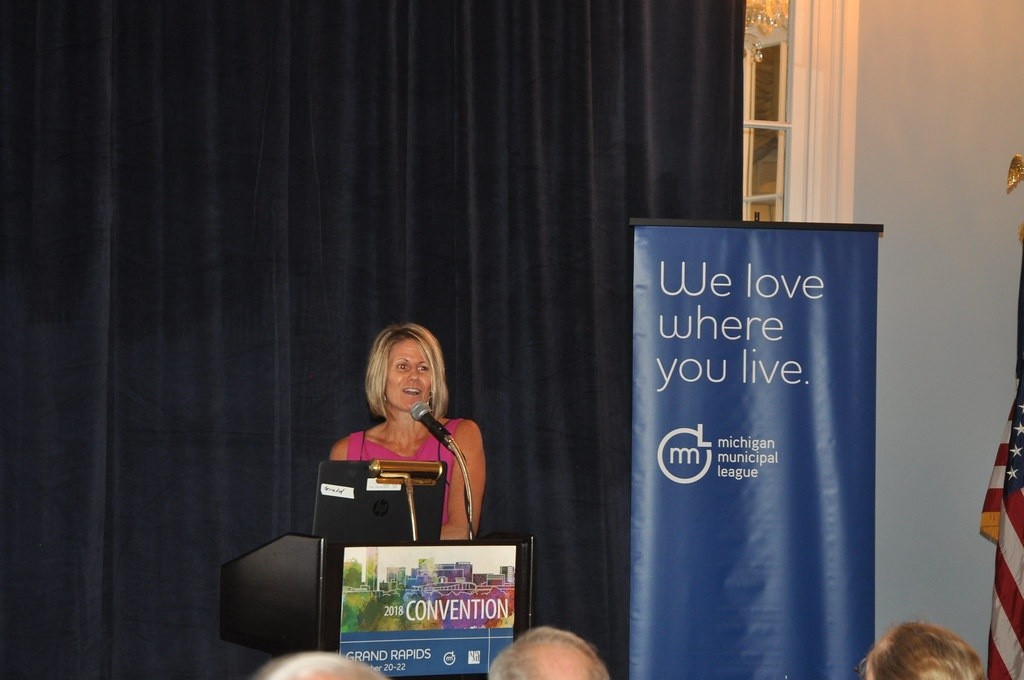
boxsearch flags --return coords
[979,372,1024,680]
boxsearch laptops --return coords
[313,460,447,542]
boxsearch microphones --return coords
[410,400,455,444]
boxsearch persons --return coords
[866,621,988,680]
[329,322,486,544]
[251,649,394,680]
[484,623,609,679]
